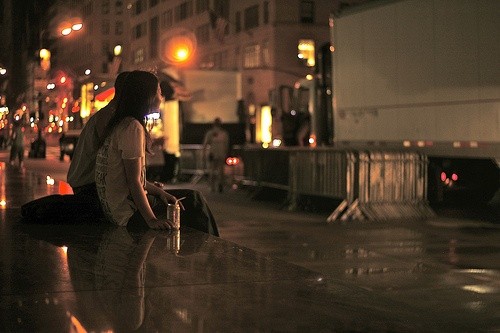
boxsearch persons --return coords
[201,117,230,194]
[69,70,221,239]
[7,119,25,168]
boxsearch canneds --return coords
[167,204,180,229]
